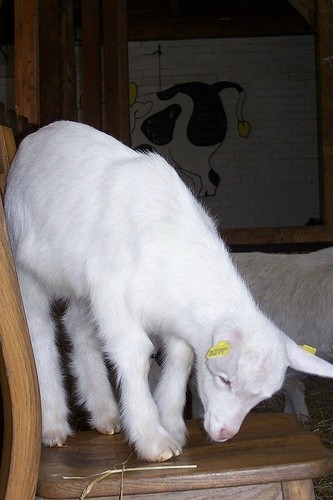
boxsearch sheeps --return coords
[1,118,333,464]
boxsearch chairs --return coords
[0,125,332,500]
